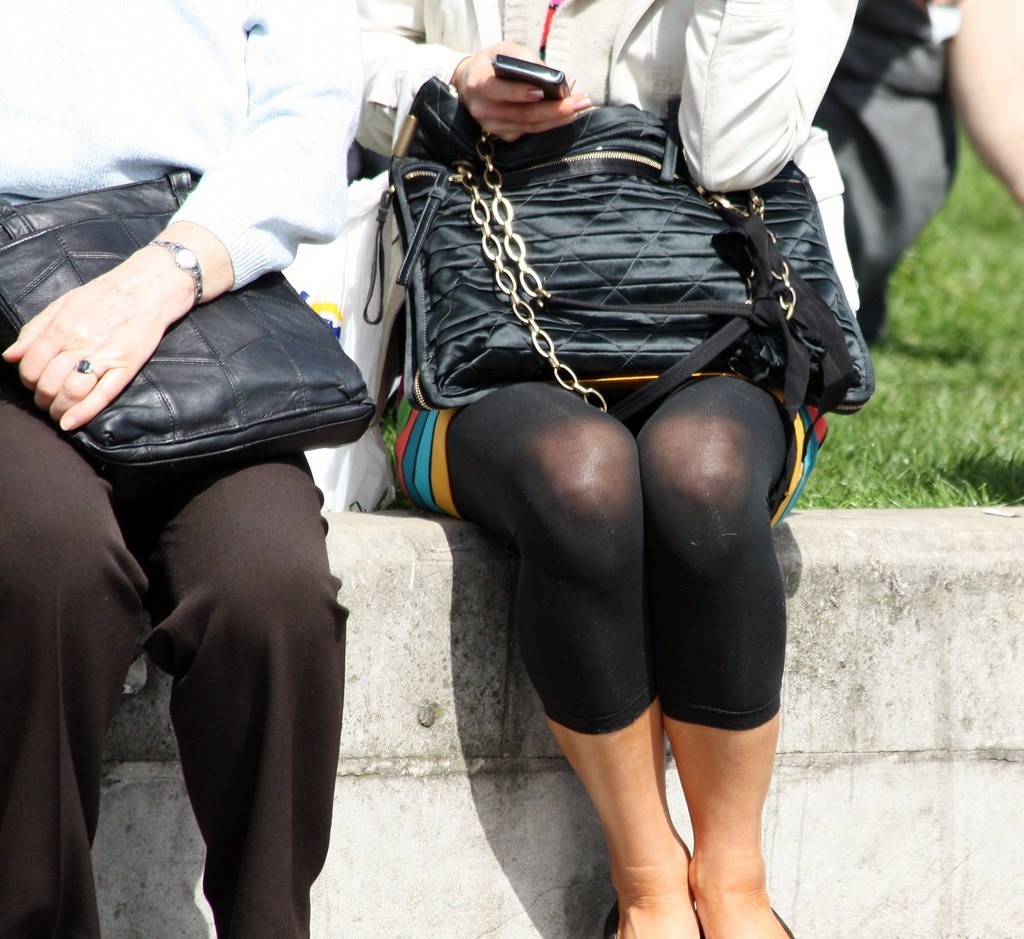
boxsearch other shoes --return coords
[604,897,795,939]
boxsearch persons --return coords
[0,0,372,938]
[810,1,1024,343]
[356,0,861,939]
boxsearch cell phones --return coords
[492,54,572,103]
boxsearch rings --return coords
[77,360,100,380]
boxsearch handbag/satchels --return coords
[363,77,876,413]
[0,169,378,468]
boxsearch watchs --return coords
[151,239,202,304]
